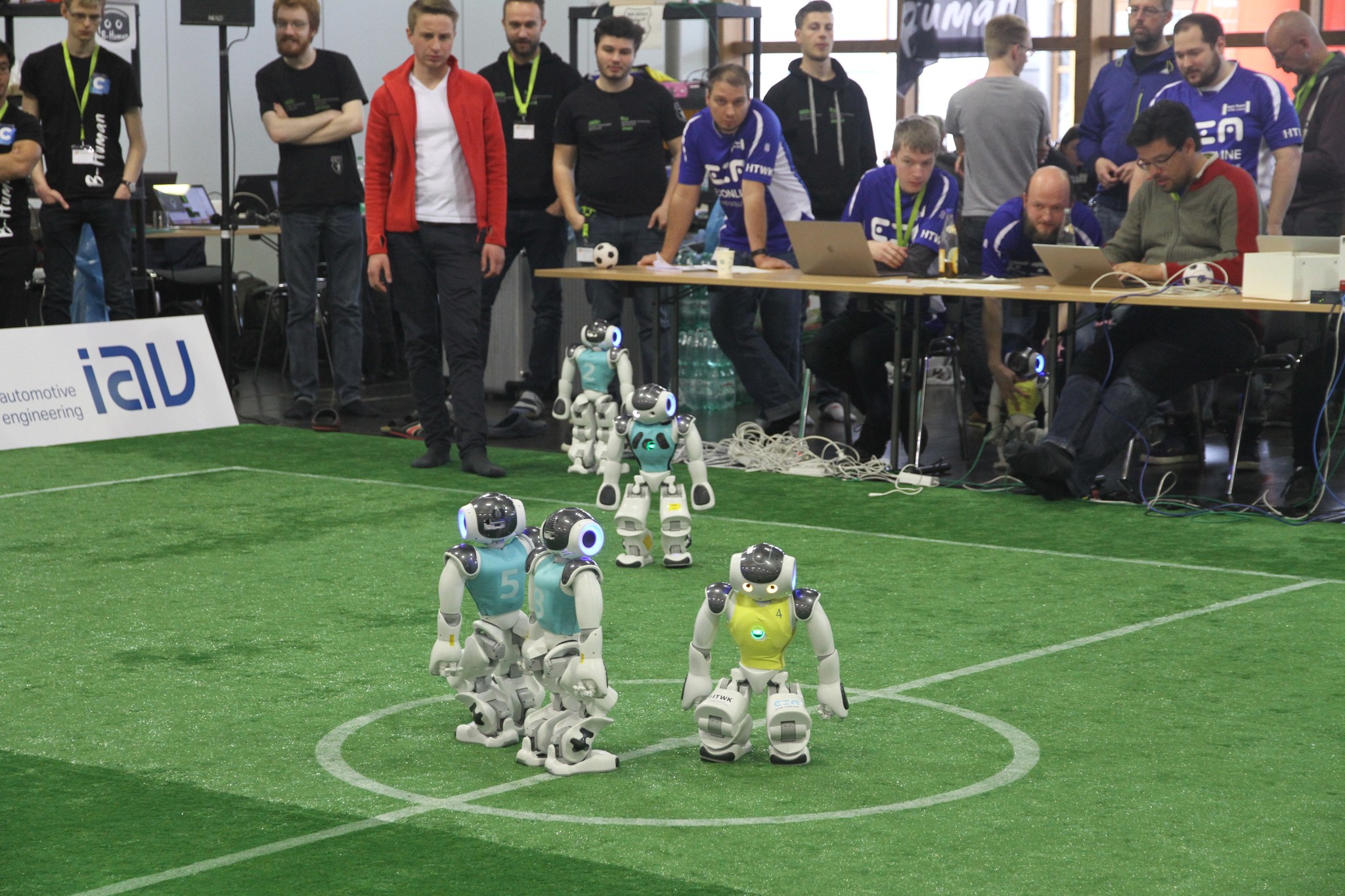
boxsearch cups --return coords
[715,250,736,275]
[153,210,170,229]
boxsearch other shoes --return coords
[1281,468,1345,523]
[338,400,380,416]
[283,393,315,419]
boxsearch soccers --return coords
[1182,261,1215,289]
[592,241,619,270]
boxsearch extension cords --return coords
[899,471,940,488]
[788,466,826,477]
[728,446,752,467]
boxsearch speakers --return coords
[180,0,257,26]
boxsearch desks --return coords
[534,265,1345,525]
[128,224,282,376]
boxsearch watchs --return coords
[120,178,136,193]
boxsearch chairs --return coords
[1120,309,1307,496]
[788,290,969,476]
[132,237,251,373]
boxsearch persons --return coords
[982,165,1106,411]
[637,64,815,439]
[762,0,878,421]
[0,0,147,331]
[365,0,507,477]
[551,318,633,475]
[551,14,688,394]
[825,115,957,465]
[883,0,1345,524]
[987,346,1050,468]
[255,0,383,420]
[1005,100,1269,499]
[595,383,715,567]
[445,0,580,426]
[430,491,847,776]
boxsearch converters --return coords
[1310,290,1345,304]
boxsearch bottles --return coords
[939,209,958,278]
[674,251,737,444]
[356,156,365,213]
[1057,208,1076,246]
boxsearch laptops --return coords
[151,182,261,229]
[784,220,923,277]
[1254,233,1342,255]
[1032,243,1153,289]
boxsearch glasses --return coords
[65,4,101,22]
[1136,145,1181,170]
[1011,42,1035,57]
[1126,8,1167,16]
[278,18,310,29]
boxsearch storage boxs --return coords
[1242,251,1341,302]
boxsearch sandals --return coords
[445,394,455,412]
[509,390,545,419]
